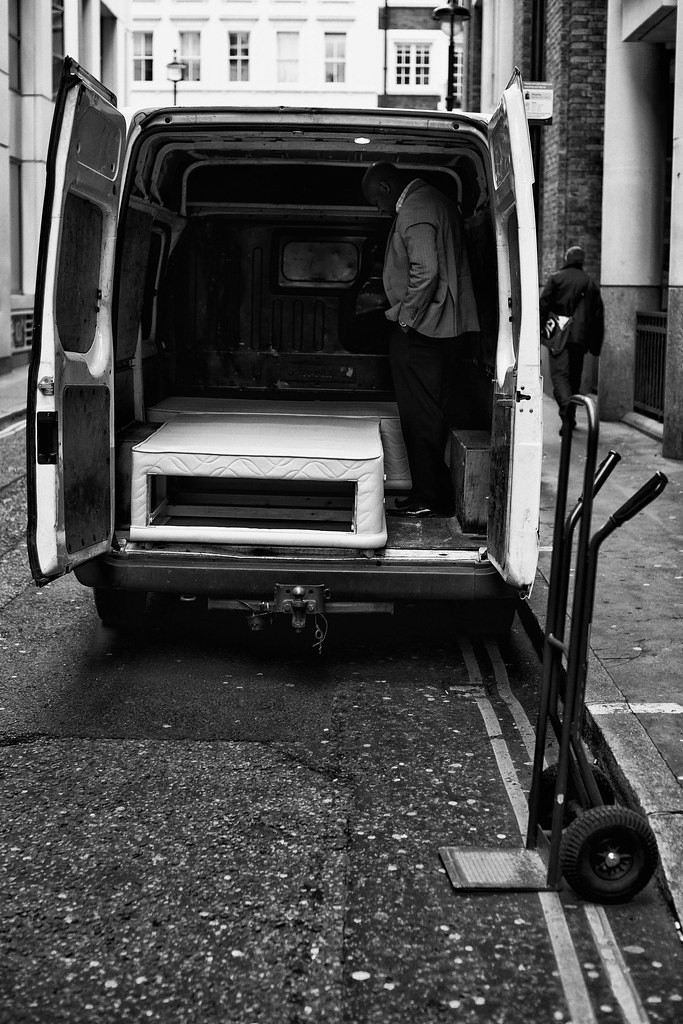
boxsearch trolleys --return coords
[440,393,670,908]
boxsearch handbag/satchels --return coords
[540,311,573,355]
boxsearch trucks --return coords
[27,56,544,655]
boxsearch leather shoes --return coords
[387,497,453,517]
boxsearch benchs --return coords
[118,398,491,556]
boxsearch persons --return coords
[367,161,478,517]
[538,245,604,436]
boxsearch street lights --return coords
[432,0,472,112]
[166,50,188,106]
